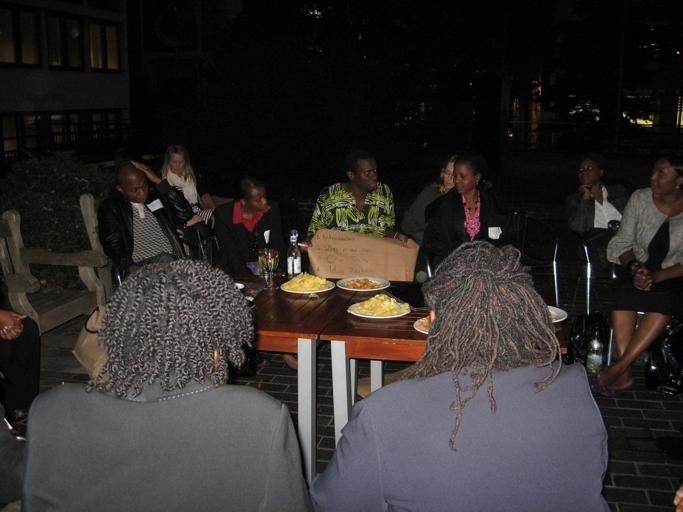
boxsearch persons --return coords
[0,262,41,441]
[671,482,683,512]
[424,154,498,274]
[564,151,629,272]
[309,239,609,512]
[214,173,284,275]
[22,258,311,512]
[595,151,683,389]
[306,150,395,238]
[161,144,216,259]
[97,160,194,275]
[399,154,464,241]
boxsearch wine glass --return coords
[263,249,280,290]
[258,251,269,290]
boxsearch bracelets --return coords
[629,261,644,277]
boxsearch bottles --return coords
[585,322,605,374]
[286,231,303,278]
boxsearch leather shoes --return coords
[590,369,639,397]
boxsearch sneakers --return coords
[3,410,29,441]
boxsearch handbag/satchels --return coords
[643,316,682,395]
[563,310,616,377]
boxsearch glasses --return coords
[575,164,599,176]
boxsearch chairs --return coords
[571,228,616,367]
[495,211,560,309]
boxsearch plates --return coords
[278,279,336,295]
[336,275,392,292]
[413,316,431,335]
[546,303,569,325]
[346,302,412,319]
